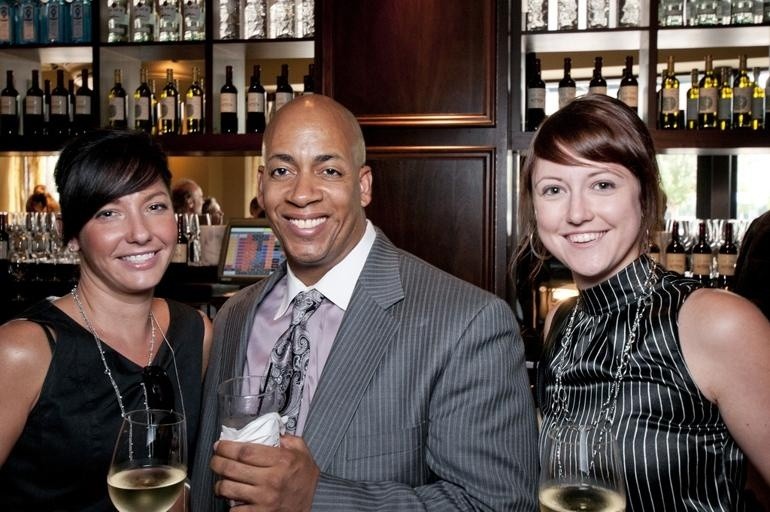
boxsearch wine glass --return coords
[107,409,189,512]
[703,218,729,280]
[10,212,199,266]
[652,218,674,271]
[676,219,703,279]
[728,220,754,268]
[538,423,627,511]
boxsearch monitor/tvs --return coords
[217,218,287,280]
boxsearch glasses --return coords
[142,365,177,465]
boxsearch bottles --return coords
[275,63,293,111]
[526,1,770,130]
[0,213,13,277]
[108,68,127,130]
[48,69,75,125]
[220,65,239,133]
[302,63,313,94]
[107,0,206,44]
[158,68,183,136]
[186,64,205,135]
[214,1,315,40]
[1,69,21,133]
[22,69,51,131]
[136,65,157,134]
[245,66,267,134]
[76,68,92,127]
[2,0,91,43]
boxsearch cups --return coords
[200,225,230,265]
[218,376,279,509]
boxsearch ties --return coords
[255,290,323,435]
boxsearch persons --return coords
[187,91,543,510]
[25,182,59,214]
[0,124,211,509]
[515,90,770,512]
[249,196,266,219]
[202,196,225,225]
[171,178,205,213]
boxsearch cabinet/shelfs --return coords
[513,24,769,413]
[0,37,315,327]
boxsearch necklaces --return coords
[548,258,660,491]
[67,284,158,465]
